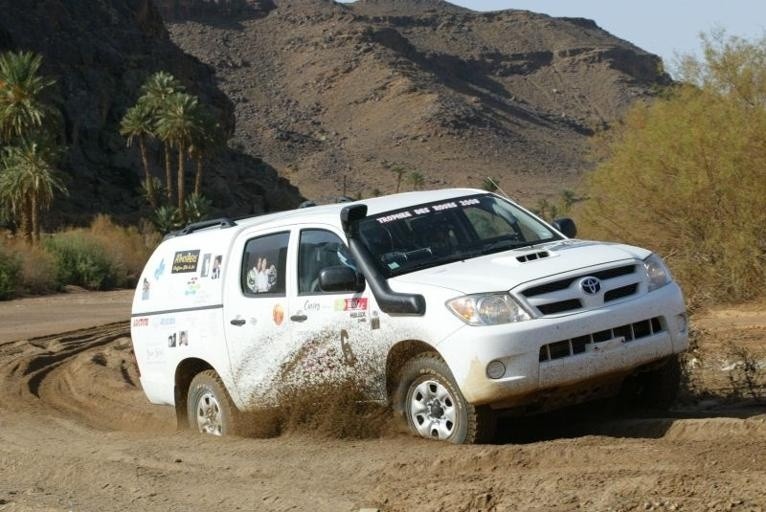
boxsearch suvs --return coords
[128,173,691,444]
